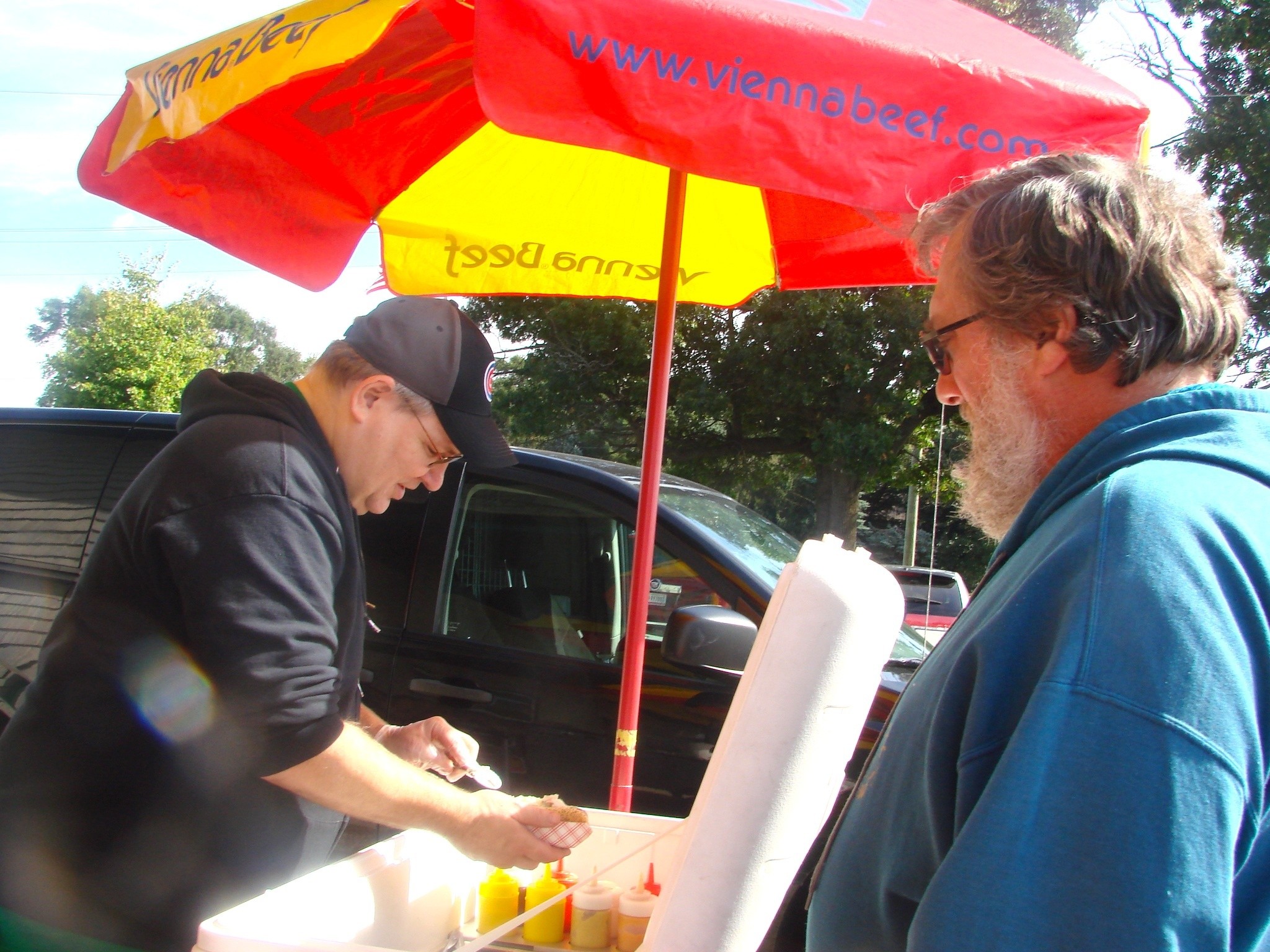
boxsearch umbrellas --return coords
[75,1,1155,816]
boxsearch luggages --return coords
[190,530,906,952]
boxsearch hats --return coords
[342,293,519,470]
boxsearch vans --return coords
[0,401,934,817]
[874,564,969,646]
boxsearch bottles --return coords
[478,857,661,952]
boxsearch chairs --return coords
[447,573,504,645]
[488,532,595,661]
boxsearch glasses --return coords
[918,308,1000,377]
[393,383,462,468]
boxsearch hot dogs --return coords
[471,789,591,849]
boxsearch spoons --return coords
[452,762,503,789]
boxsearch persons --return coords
[2,297,577,950]
[794,148,1266,951]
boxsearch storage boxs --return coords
[189,533,908,951]
